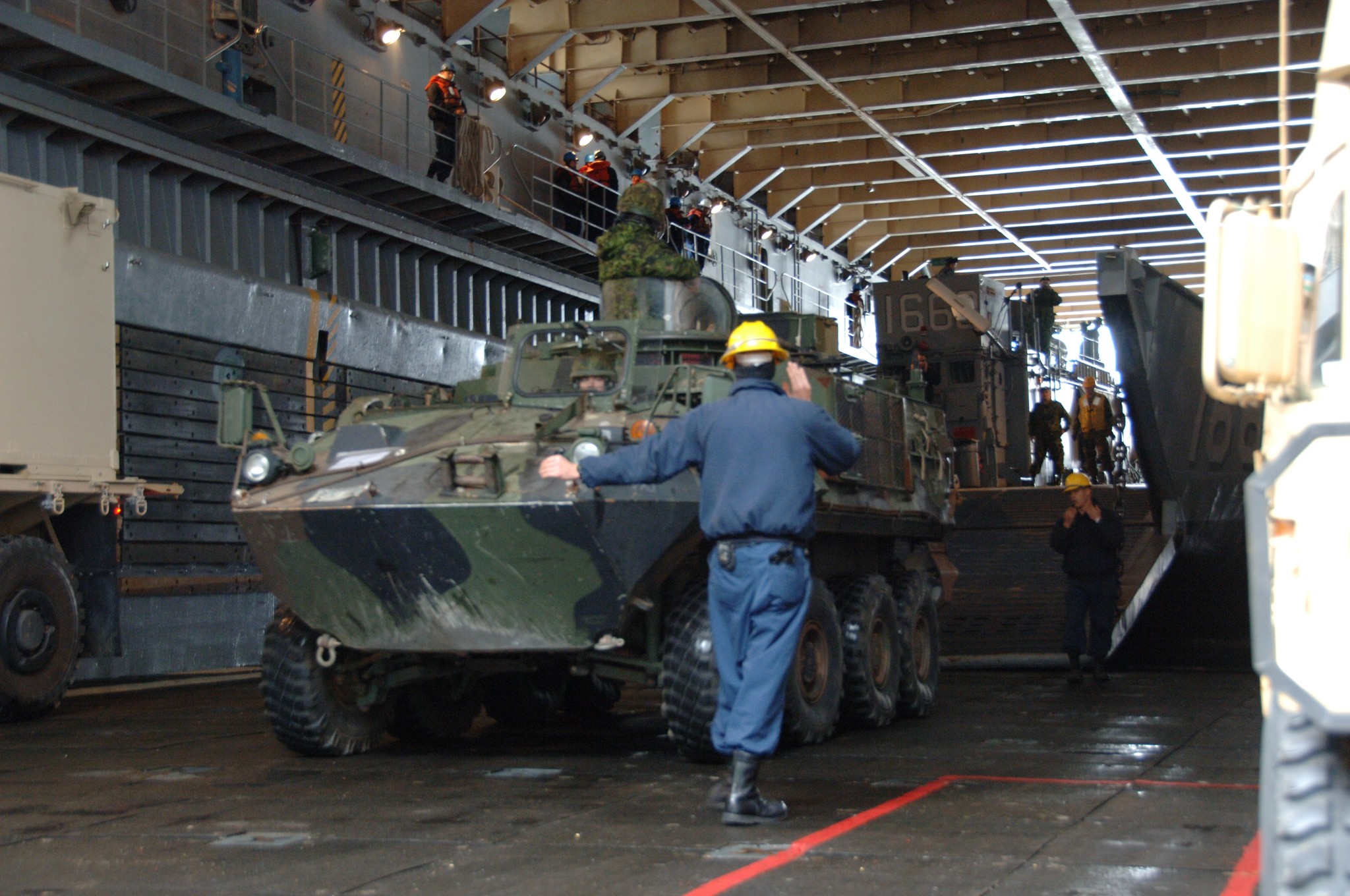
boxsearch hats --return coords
[1040,277,1050,281]
[1041,386,1049,390]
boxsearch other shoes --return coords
[1025,471,1121,486]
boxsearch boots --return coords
[720,750,788,825]
[1091,656,1110,691]
[715,758,732,810]
[1065,650,1083,682]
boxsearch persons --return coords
[1085,317,1102,361]
[1026,276,1062,357]
[936,257,958,280]
[578,154,595,239]
[537,321,861,825]
[686,199,712,272]
[553,151,582,236]
[424,63,468,183]
[582,149,619,244]
[630,169,643,184]
[1048,473,1127,687]
[1071,376,1115,485]
[662,197,691,256]
[844,288,864,349]
[1028,387,1071,486]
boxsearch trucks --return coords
[0,170,190,721]
[1196,0,1350,892]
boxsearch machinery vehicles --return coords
[210,274,956,763]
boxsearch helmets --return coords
[569,351,617,380]
[617,182,664,224]
[1063,472,1092,493]
[852,284,862,290]
[563,148,712,210]
[1093,318,1103,325]
[440,62,456,73]
[1083,376,1096,388]
[719,321,789,371]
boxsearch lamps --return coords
[800,247,817,262]
[835,268,853,282]
[853,278,870,291]
[710,200,723,214]
[363,20,405,46]
[775,237,794,252]
[527,103,551,127]
[570,123,593,147]
[626,159,650,177]
[754,225,773,240]
[478,81,506,103]
[673,182,694,199]
